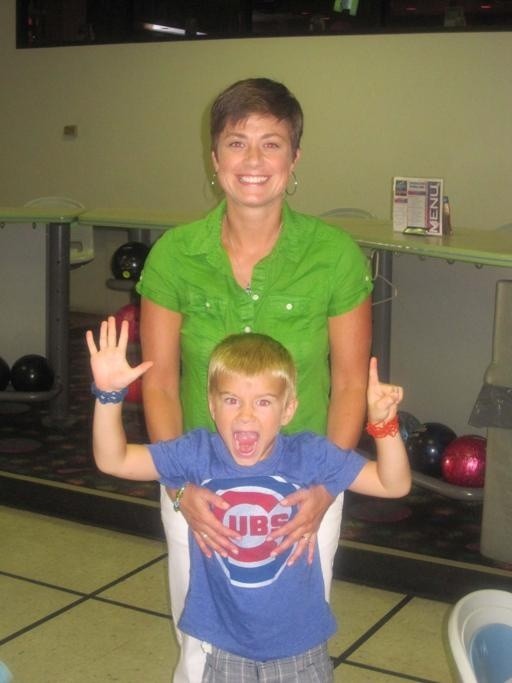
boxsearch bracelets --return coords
[173,482,186,512]
[365,414,400,438]
[90,383,128,405]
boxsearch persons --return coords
[134,78,374,683]
[86,314,412,683]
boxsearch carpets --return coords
[0,338,512,605]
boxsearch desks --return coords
[284,204,511,497]
[0,204,214,499]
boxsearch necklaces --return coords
[225,223,281,295]
